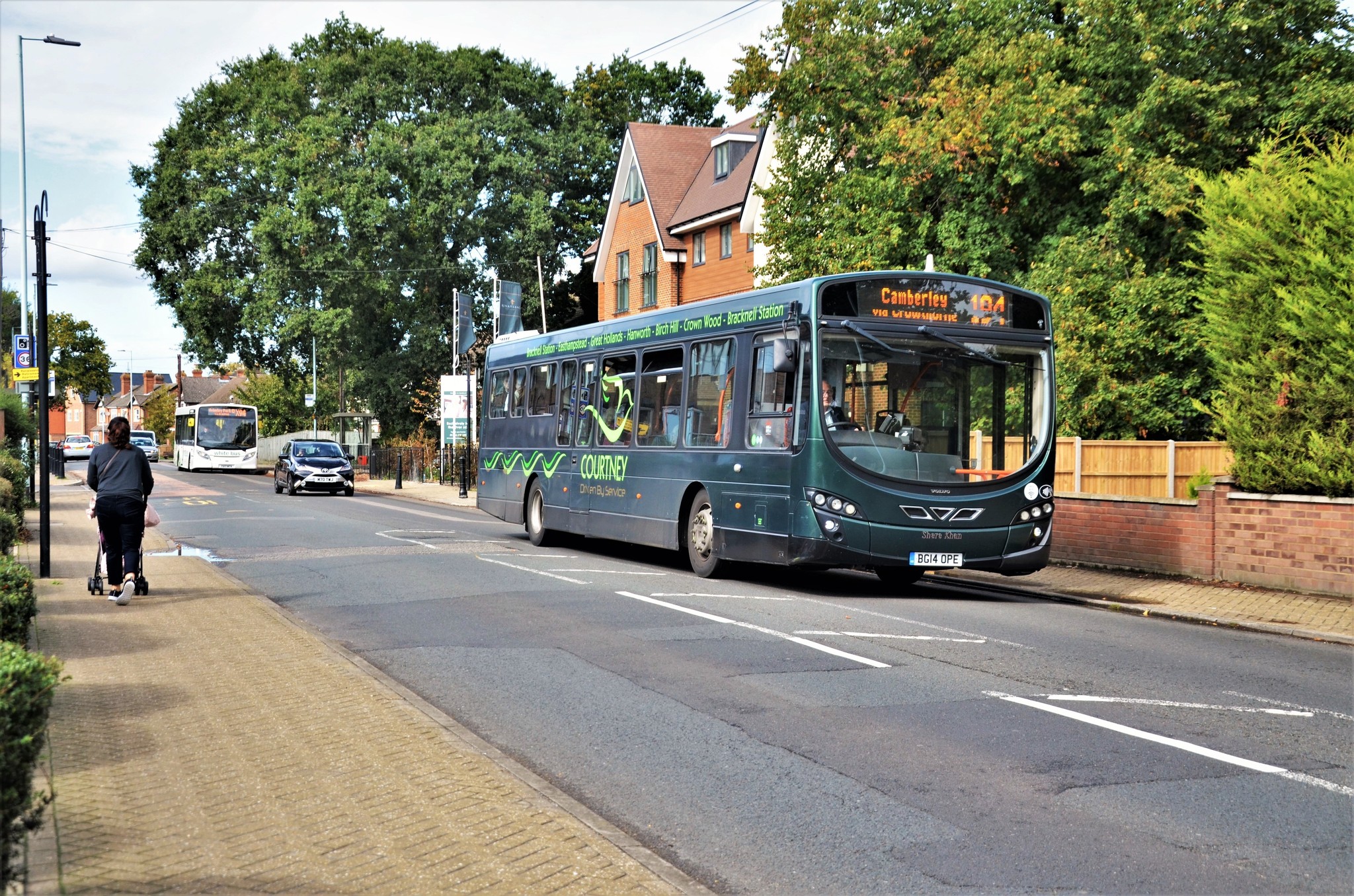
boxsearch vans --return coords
[129,430,160,448]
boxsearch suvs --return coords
[274,438,356,497]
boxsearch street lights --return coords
[18,33,81,498]
[117,349,133,430]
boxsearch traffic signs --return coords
[12,366,50,382]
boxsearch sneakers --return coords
[108,588,121,600]
[115,576,135,605]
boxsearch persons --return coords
[799,380,865,434]
[86,417,155,606]
[492,373,524,413]
[296,448,306,456]
[200,426,215,437]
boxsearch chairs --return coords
[784,407,793,444]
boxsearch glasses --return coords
[822,391,834,396]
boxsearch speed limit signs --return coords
[17,352,30,367]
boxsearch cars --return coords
[92,441,102,448]
[48,441,65,449]
[129,436,160,463]
[59,435,95,463]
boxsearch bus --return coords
[173,404,258,474]
[475,270,1058,588]
